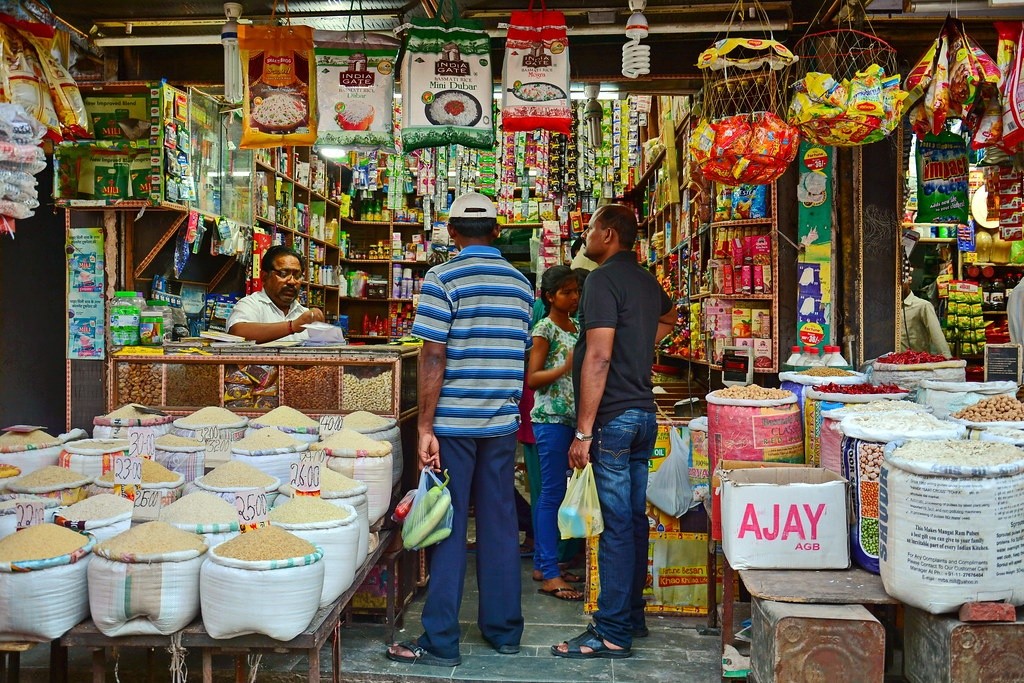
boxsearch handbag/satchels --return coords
[557,461,604,540]
[389,464,453,551]
[645,424,693,519]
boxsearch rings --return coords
[312,311,315,318]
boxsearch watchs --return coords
[575,429,593,442]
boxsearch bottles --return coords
[249,130,340,328]
[359,197,390,222]
[788,345,849,366]
[110,291,173,345]
[920,225,1024,310]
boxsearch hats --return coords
[449,192,498,219]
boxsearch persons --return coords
[550,204,676,659]
[226,245,325,345]
[386,192,534,668]
[517,235,599,602]
[901,255,953,360]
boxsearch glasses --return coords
[271,269,305,283]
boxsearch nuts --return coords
[116,362,222,409]
[284,365,394,411]
[713,384,792,400]
[858,444,887,483]
[951,395,1024,422]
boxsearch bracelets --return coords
[288,320,294,334]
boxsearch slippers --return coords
[466,541,476,554]
[385,640,520,667]
[532,572,585,602]
[550,622,649,659]
[518,541,536,556]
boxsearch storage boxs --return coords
[699,226,851,571]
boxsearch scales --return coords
[720,345,754,387]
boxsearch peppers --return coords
[812,380,910,395]
[876,348,961,364]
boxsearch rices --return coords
[430,92,479,126]
[341,101,374,126]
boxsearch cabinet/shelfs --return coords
[66,89,797,373]
